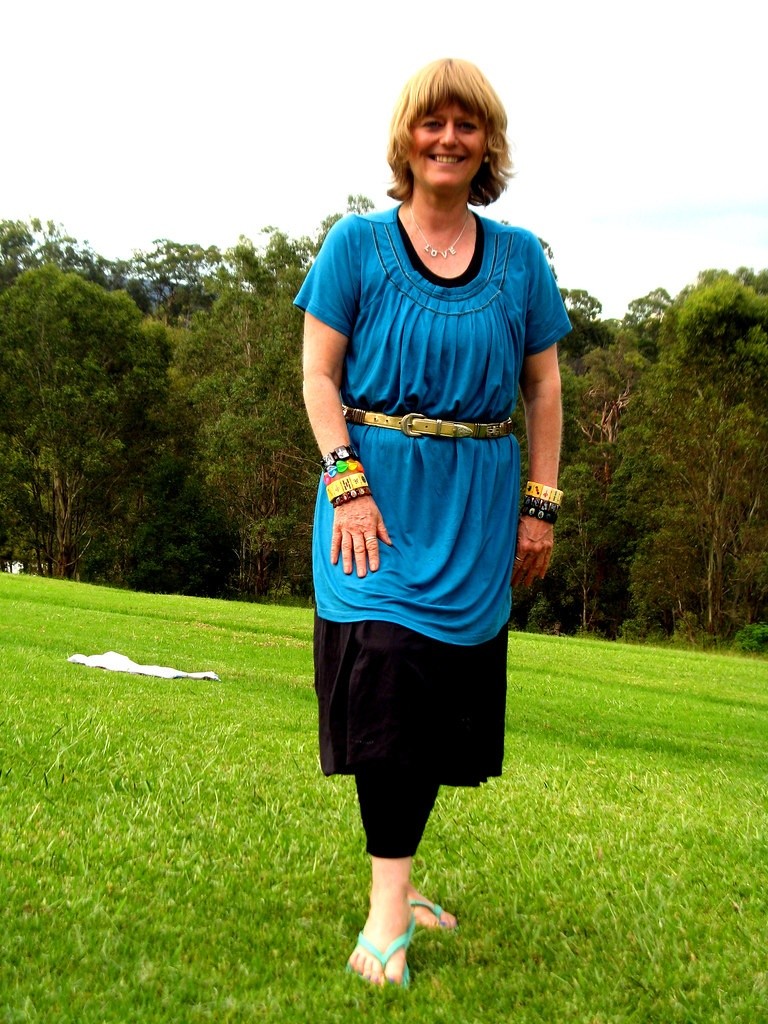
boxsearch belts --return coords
[341,405,514,438]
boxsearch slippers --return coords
[346,910,415,993]
[409,900,444,930]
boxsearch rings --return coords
[365,537,377,541]
[515,556,524,562]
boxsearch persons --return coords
[292,59,571,987]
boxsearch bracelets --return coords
[525,480,564,505]
[520,497,560,524]
[320,444,364,485]
[326,473,372,507]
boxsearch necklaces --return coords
[409,204,470,258]
